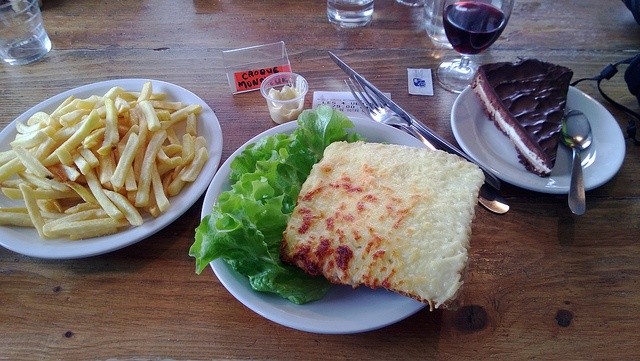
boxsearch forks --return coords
[343,73,511,215]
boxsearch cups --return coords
[258,71,309,125]
[423,0,453,48]
[0,0,52,67]
[327,0,374,28]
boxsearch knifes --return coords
[325,50,502,192]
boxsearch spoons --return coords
[559,109,594,216]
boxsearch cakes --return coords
[469,58,574,177]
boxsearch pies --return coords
[278,140,485,310]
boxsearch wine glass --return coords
[435,0,514,94]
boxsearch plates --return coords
[0,78,224,261]
[200,116,430,335]
[450,84,626,195]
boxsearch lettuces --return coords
[189,103,366,306]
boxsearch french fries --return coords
[0,81,209,240]
